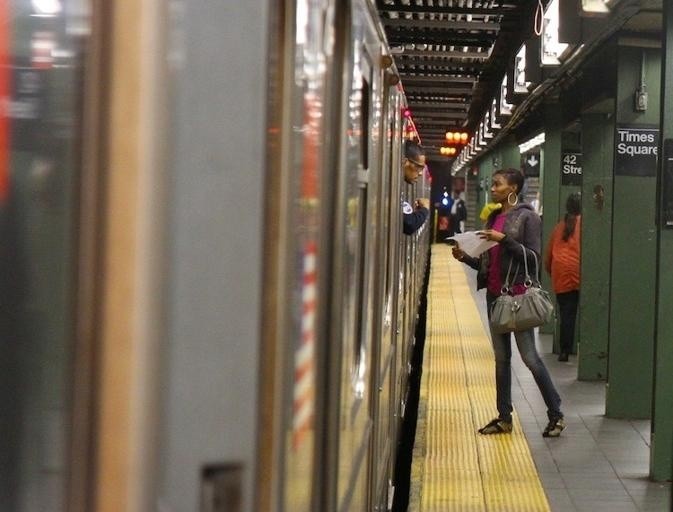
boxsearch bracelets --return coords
[454,253,464,261]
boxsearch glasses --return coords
[409,159,425,172]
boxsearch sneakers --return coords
[477,417,512,435]
[542,415,568,438]
[558,353,569,361]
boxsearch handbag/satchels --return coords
[488,244,556,337]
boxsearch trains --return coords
[0,0,432,512]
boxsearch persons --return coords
[529,192,543,220]
[446,189,466,245]
[402,138,430,235]
[544,190,584,361]
[449,169,568,439]
